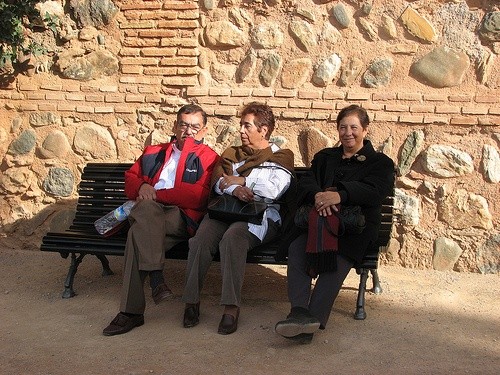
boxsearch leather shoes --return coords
[217,308,239,334]
[152,282,173,304]
[183,306,199,327]
[103,313,144,335]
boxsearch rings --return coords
[243,195,245,198]
[224,183,227,186]
[321,202,323,205]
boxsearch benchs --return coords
[39,164,399,320]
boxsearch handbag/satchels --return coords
[305,205,345,252]
[207,192,268,225]
[294,201,366,234]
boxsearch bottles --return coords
[94,200,138,235]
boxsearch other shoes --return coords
[275,313,320,337]
[282,333,313,343]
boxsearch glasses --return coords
[175,123,205,134]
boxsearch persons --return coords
[182,101,297,336]
[275,105,391,344]
[102,104,220,335]
